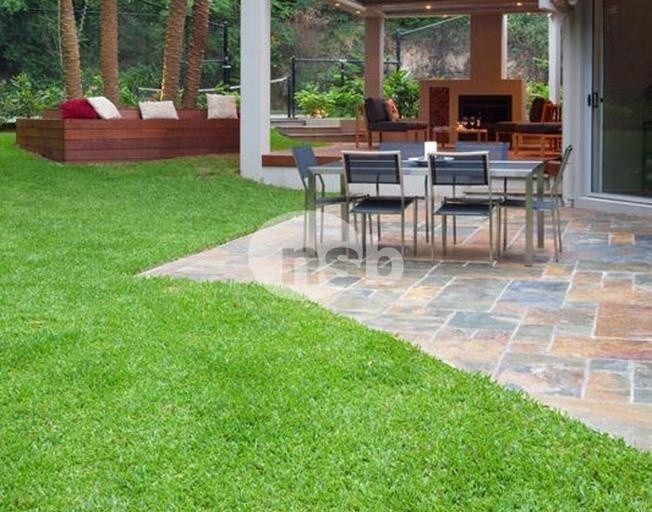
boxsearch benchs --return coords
[16,107,239,165]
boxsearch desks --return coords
[308,159,544,267]
[434,125,489,143]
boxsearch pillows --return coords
[138,100,179,119]
[60,98,98,119]
[385,99,400,122]
[87,96,124,119]
[528,96,546,123]
[205,93,239,120]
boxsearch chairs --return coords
[375,139,429,246]
[426,151,506,268]
[501,144,573,264]
[340,150,417,258]
[492,103,562,162]
[292,144,365,251]
[363,95,428,142]
[451,141,509,253]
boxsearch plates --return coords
[408,155,455,166]
